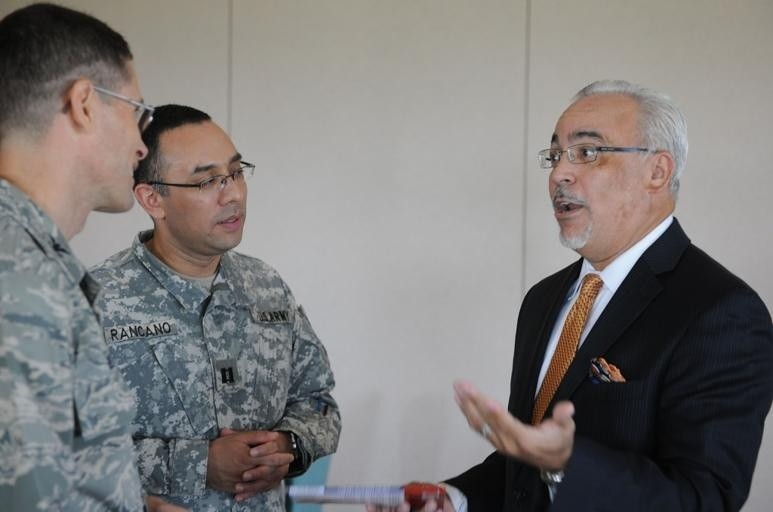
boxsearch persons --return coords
[1,1,189,512]
[86,104,342,512]
[364,78,773,512]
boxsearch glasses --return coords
[540,144,663,170]
[60,85,154,131]
[132,162,255,196]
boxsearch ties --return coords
[533,274,603,426]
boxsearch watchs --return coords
[290,432,298,459]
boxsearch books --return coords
[288,484,445,512]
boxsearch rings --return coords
[479,423,492,439]
